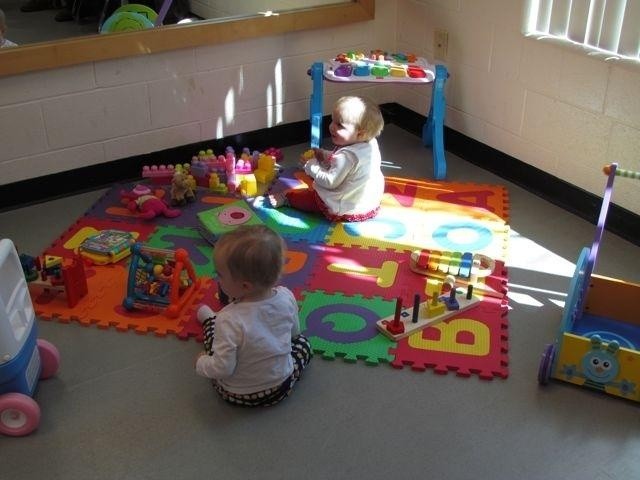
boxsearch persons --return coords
[268,94,385,224]
[0,9,19,49]
[194,224,312,410]
